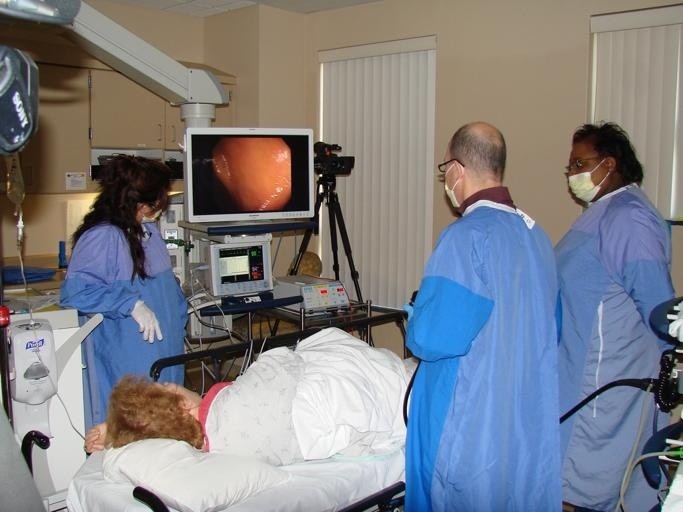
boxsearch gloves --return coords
[131,300,163,344]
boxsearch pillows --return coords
[102,438,291,512]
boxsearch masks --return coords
[567,172,600,201]
[444,177,459,209]
[139,209,163,223]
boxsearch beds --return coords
[20,310,405,512]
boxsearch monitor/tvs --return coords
[183,127,316,224]
[207,241,274,296]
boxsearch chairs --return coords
[19,62,90,194]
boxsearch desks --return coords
[0,254,70,295]
[178,220,317,292]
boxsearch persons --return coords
[59,151,188,435]
[84,372,235,453]
[550,120,682,511]
[402,121,563,512]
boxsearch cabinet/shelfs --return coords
[90,61,237,151]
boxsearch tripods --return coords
[271,176,375,347]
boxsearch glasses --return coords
[437,159,464,174]
[563,155,602,168]
